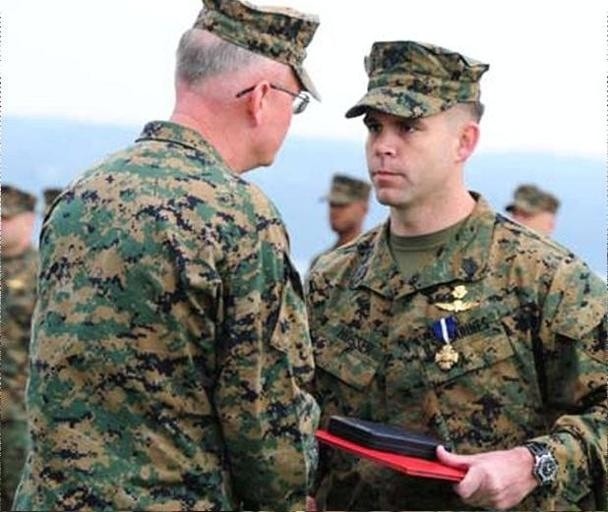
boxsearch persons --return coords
[503,183,560,239]
[1,185,40,511]
[309,175,372,267]
[9,0,322,512]
[42,186,61,210]
[298,41,608,512]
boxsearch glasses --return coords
[234,81,311,114]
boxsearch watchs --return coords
[527,440,558,489]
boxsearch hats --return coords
[41,188,61,216]
[345,39,491,120]
[505,184,561,217]
[190,0,324,103]
[1,184,37,220]
[319,174,373,211]
